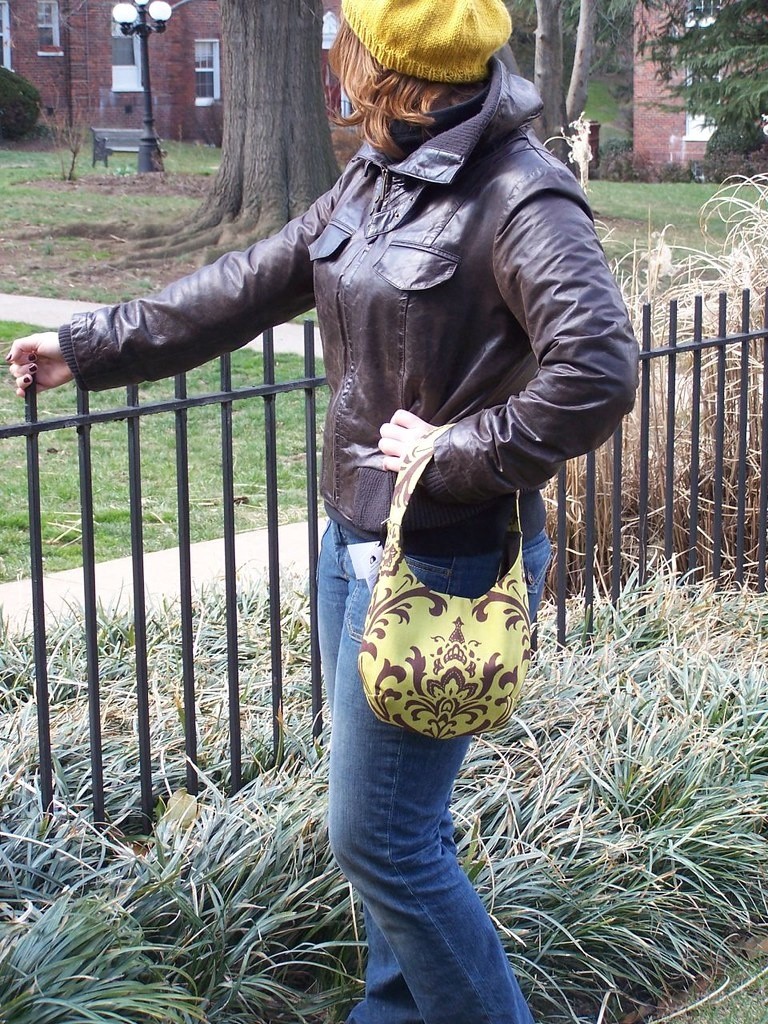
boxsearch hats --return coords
[341,0,512,83]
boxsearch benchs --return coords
[90,127,148,168]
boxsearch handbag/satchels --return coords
[358,424,530,740]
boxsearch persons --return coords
[7,0,640,1024]
